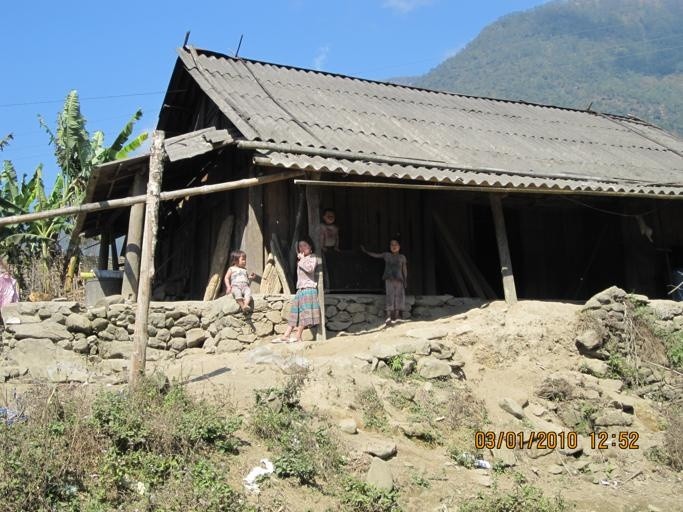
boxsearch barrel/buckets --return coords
[85,275,122,308]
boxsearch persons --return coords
[222,250,257,312]
[358,237,407,324]
[317,208,341,294]
[268,234,320,343]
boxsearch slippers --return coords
[272,338,297,343]
